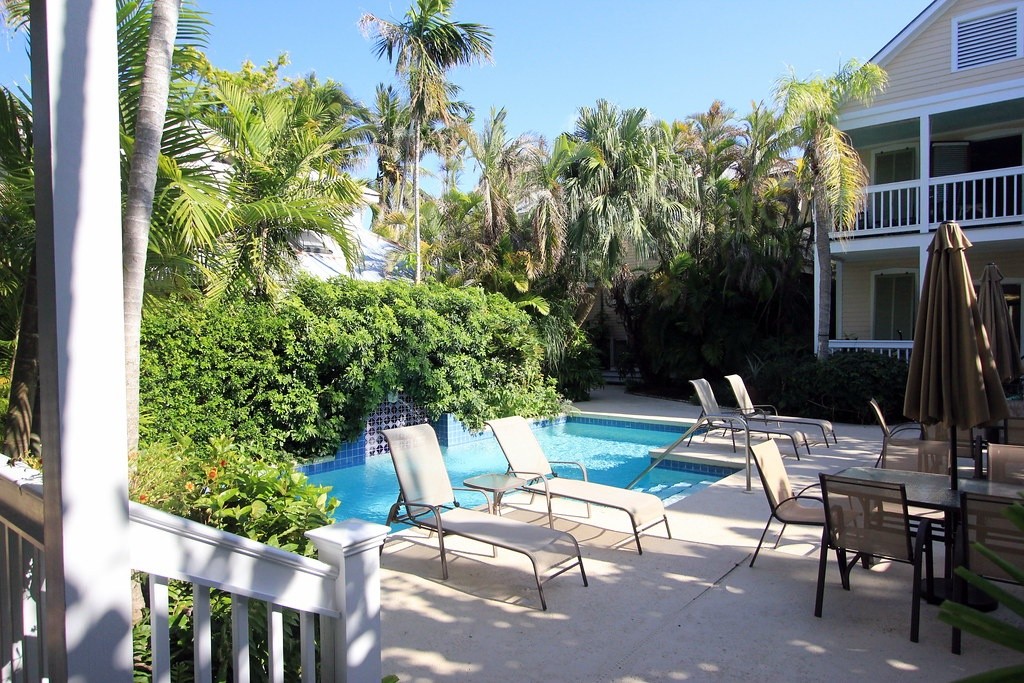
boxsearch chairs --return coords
[686,377,811,460]
[921,420,984,479]
[1003,416,1024,479]
[379,424,588,611]
[951,489,1024,655]
[815,473,934,643]
[973,435,1024,581]
[483,415,672,556]
[876,424,958,579]
[870,398,929,473]
[748,439,861,569]
[722,374,839,448]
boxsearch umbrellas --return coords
[978,262,1024,444]
[903,220,1013,490]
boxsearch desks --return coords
[463,473,525,556]
[825,466,1024,615]
[973,419,1024,479]
